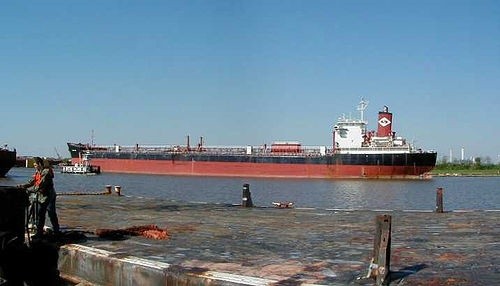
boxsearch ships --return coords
[65,97,437,179]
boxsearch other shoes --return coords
[31,233,45,241]
[48,236,60,243]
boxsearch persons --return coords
[16,156,61,239]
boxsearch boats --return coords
[61,150,101,176]
